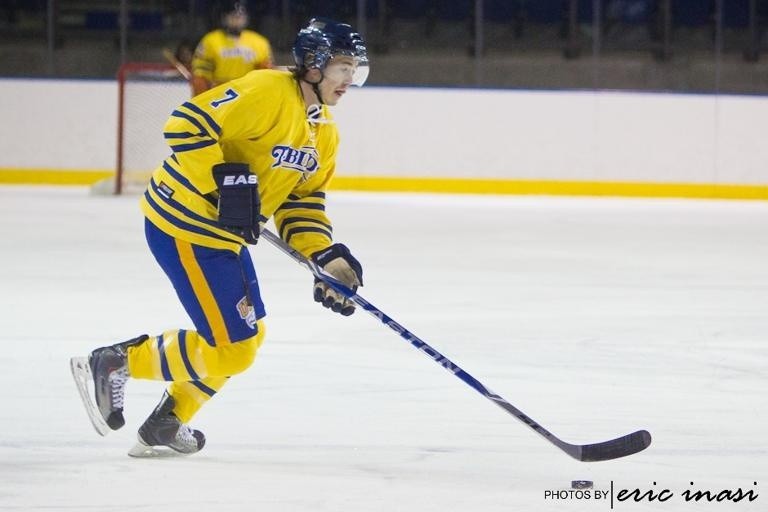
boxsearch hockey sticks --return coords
[257,223,650,461]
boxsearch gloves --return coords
[311,242,364,317]
[212,162,261,245]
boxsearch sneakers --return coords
[137,388,205,454]
[89,334,149,430]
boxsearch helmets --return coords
[294,16,370,105]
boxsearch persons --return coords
[190,0,273,97]
[87,16,371,457]
